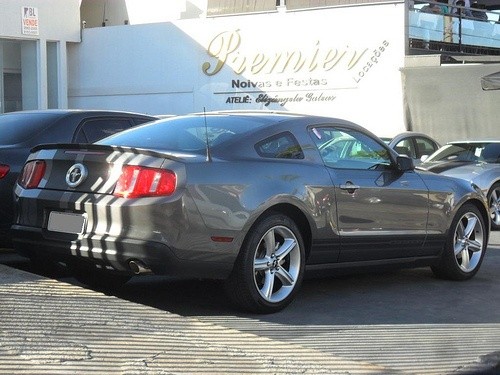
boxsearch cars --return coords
[11,109,493,321]
[313,132,442,171]
[0,105,162,256]
[411,137,500,233]
